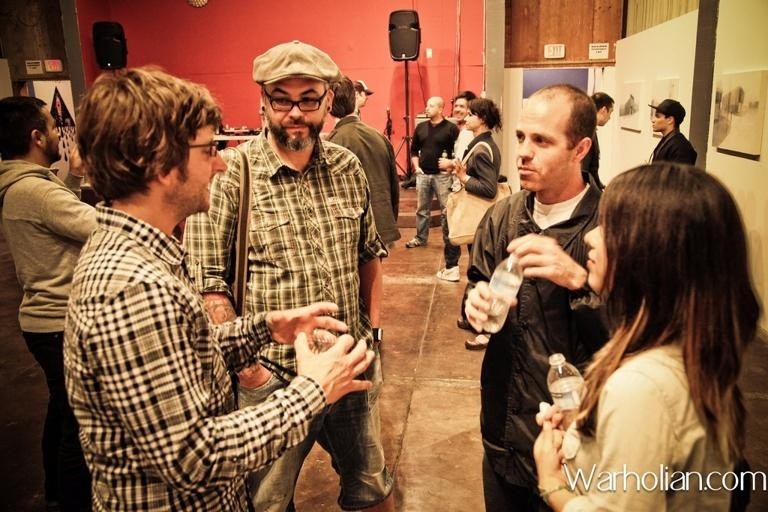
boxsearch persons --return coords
[62,66,375,512]
[404,83,768,512]
[0,94,100,511]
[181,41,394,512]
[325,75,401,247]
[335,80,375,123]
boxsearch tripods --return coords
[394,61,413,180]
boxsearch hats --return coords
[252,40,340,85]
[352,80,373,94]
[648,99,684,122]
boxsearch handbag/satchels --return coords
[445,183,511,247]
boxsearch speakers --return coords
[93,21,127,69]
[389,10,421,61]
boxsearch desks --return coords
[212,127,330,152]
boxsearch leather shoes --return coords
[465,339,485,350]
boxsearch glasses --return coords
[263,89,326,111]
[187,140,218,156]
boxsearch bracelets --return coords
[372,328,383,342]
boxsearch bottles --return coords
[475,252,523,335]
[440,149,449,176]
[544,351,588,433]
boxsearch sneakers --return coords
[406,237,426,248]
[436,266,460,282]
